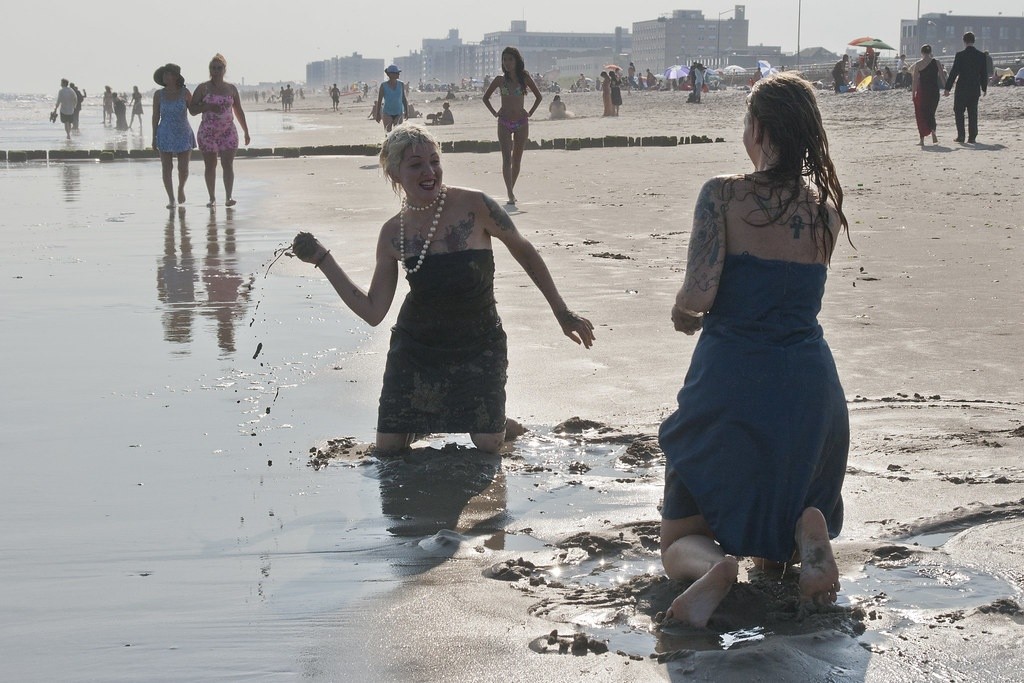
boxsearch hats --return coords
[387,65,401,73]
[154,63,185,87]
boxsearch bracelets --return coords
[315,250,330,268]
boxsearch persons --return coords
[151,64,204,209]
[483,46,542,204]
[571,62,665,92]
[656,71,849,628]
[549,95,566,118]
[292,123,596,451]
[254,83,341,111]
[753,69,763,83]
[831,31,994,146]
[353,72,562,126]
[375,64,409,137]
[672,62,707,102]
[601,71,622,116]
[53,78,144,139]
[189,53,251,207]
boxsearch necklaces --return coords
[400,183,447,274]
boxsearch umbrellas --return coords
[607,65,623,71]
[665,65,690,79]
[848,36,873,57]
[855,38,895,91]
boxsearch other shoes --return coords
[49,111,59,123]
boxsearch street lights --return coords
[716,8,743,72]
